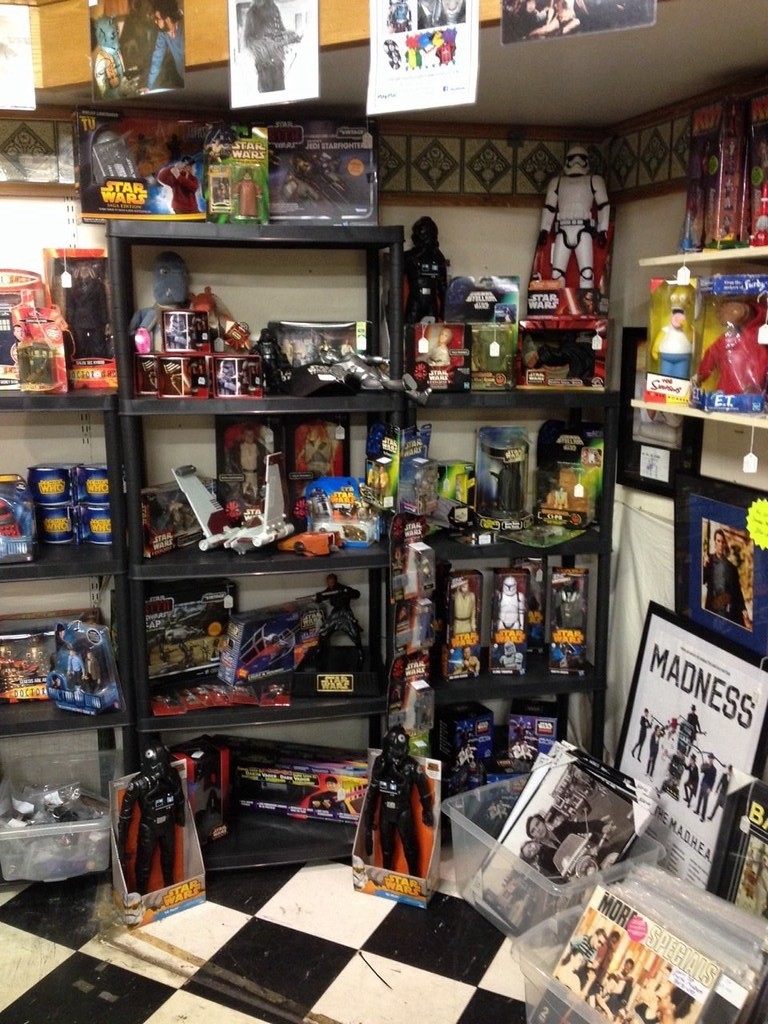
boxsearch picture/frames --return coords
[613,600,767,899]
[663,472,767,656]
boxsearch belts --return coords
[243,469,257,473]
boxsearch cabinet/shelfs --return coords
[1,210,615,875]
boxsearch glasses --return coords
[598,940,604,946]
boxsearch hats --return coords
[690,705,697,710]
[708,753,715,759]
[690,753,697,762]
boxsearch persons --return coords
[400,214,449,325]
[65,261,110,358]
[702,528,754,631]
[66,649,84,687]
[441,643,767,1019]
[313,573,366,669]
[119,737,186,895]
[309,776,348,814]
[365,723,435,878]
[414,690,430,730]
[88,116,768,643]
[84,648,102,693]
[96,0,305,98]
